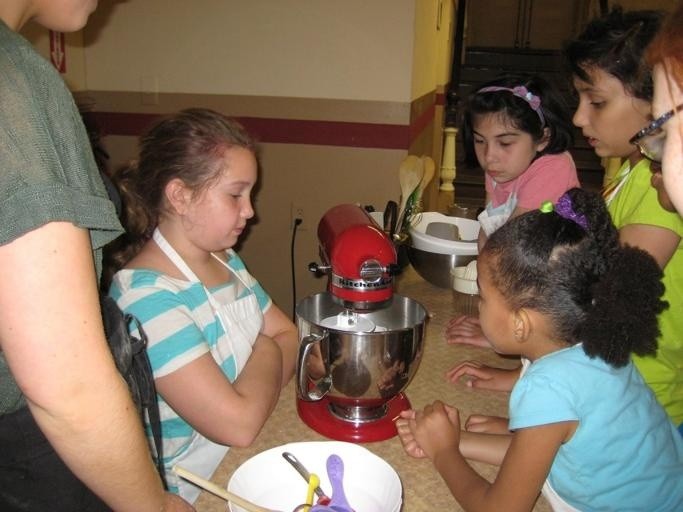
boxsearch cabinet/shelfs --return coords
[441,0,608,206]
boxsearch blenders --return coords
[295,202,429,442]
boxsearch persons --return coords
[394,187,683,512]
[2,0,194,512]
[630,0,683,217]
[443,10,683,437]
[107,107,301,505]
[446,73,581,350]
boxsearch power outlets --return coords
[292,202,307,231]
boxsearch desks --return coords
[190,261,555,512]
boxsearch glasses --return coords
[628,104,682,164]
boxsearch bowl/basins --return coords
[405,212,482,288]
[226,441,403,512]
[386,233,408,268]
